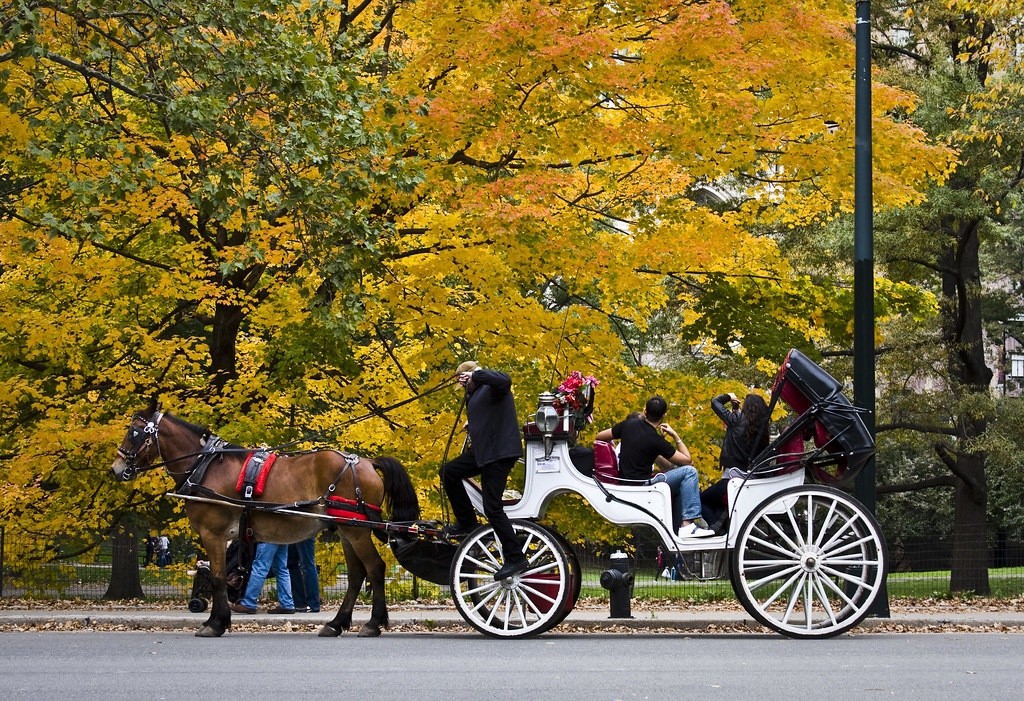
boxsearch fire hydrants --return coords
[600,549,635,619]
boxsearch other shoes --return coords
[296,608,320,612]
[266,606,295,614]
[230,604,256,614]
[708,510,729,533]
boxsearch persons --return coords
[142,529,170,567]
[187,541,231,577]
[616,411,709,529]
[654,546,669,581]
[699,393,769,535]
[595,397,716,537]
[438,361,530,581]
[289,531,320,612]
[230,543,295,614]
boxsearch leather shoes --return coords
[494,559,530,581]
[451,525,479,535]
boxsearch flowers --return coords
[539,370,600,430]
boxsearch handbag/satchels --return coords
[662,569,670,578]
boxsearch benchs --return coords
[593,439,620,484]
[522,395,576,447]
[776,425,804,473]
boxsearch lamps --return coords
[536,391,559,460]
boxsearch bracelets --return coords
[676,440,682,445]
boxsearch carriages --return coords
[107,348,890,640]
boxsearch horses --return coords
[108,393,421,637]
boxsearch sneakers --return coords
[678,523,716,538]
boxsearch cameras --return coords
[732,401,740,411]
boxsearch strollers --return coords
[188,512,257,613]
